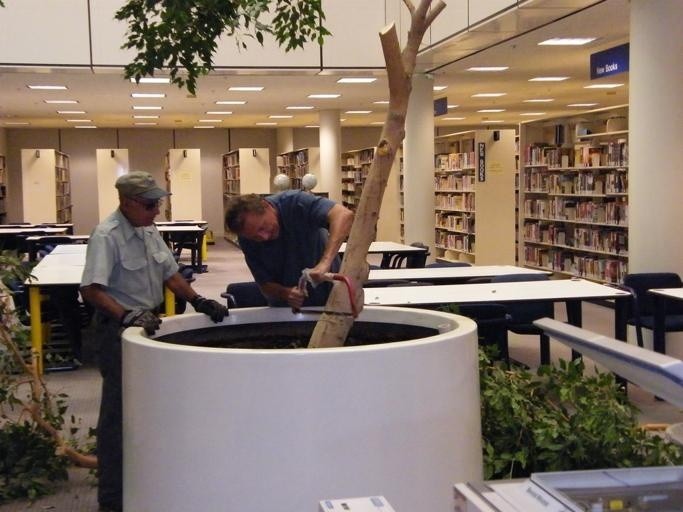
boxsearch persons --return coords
[81,170,230,512]
[224,189,355,307]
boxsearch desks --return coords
[339,240,423,272]
[644,286,682,401]
[354,276,630,380]
[0,222,210,372]
[353,262,553,365]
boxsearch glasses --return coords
[128,199,162,210]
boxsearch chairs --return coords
[620,273,682,348]
[392,239,433,268]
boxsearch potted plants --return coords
[112,0,484,508]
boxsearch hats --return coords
[115,171,172,201]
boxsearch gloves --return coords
[193,295,229,323]
[120,306,162,335]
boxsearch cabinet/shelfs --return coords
[0,153,11,225]
[434,128,516,265]
[221,147,272,250]
[516,103,630,285]
[95,148,129,224]
[162,147,203,220]
[340,147,402,241]
[277,146,323,192]
[20,149,73,223]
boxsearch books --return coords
[164,154,172,222]
[277,151,307,188]
[524,141,628,284]
[56,153,72,224]
[341,148,474,254]
[225,149,240,241]
